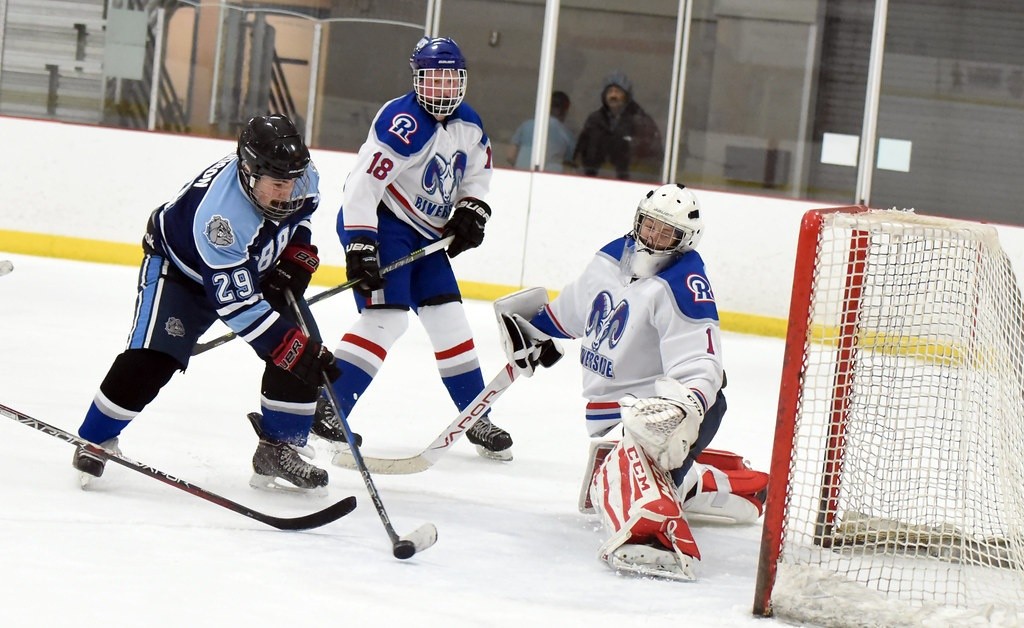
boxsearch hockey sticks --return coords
[286,287,438,554]
[190,235,455,356]
[332,362,520,476]
[0,404,357,531]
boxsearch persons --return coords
[507,90,573,172]
[492,184,769,582]
[572,72,664,184]
[73,114,343,498]
[309,36,514,461]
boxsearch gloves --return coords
[259,241,319,310]
[346,236,384,298]
[251,316,340,394]
[441,197,492,258]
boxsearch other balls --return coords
[393,541,416,559]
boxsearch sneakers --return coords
[248,412,329,496]
[309,397,363,451]
[72,447,108,491]
[466,417,513,462]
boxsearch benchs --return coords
[0,0,126,124]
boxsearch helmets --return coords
[631,183,706,280]
[409,36,467,113]
[236,113,310,221]
